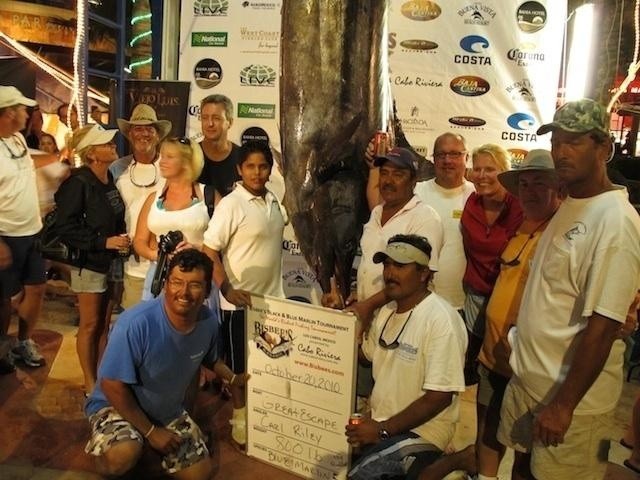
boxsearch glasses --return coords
[435,151,466,159]
[129,161,157,187]
[177,136,189,144]
[496,232,534,266]
[379,310,412,349]
[1,136,28,158]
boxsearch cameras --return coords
[159,230,184,256]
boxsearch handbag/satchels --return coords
[38,214,86,266]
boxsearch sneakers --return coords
[11,339,45,367]
[231,410,247,446]
[2,351,16,373]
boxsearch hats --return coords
[497,149,555,196]
[117,103,173,142]
[538,99,610,137]
[373,242,429,267]
[75,123,119,151]
[0,84,38,108]
[373,147,420,170]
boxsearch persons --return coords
[82,248,250,480]
[322,95,640,480]
[0,86,287,455]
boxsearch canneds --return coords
[349,413,369,447]
